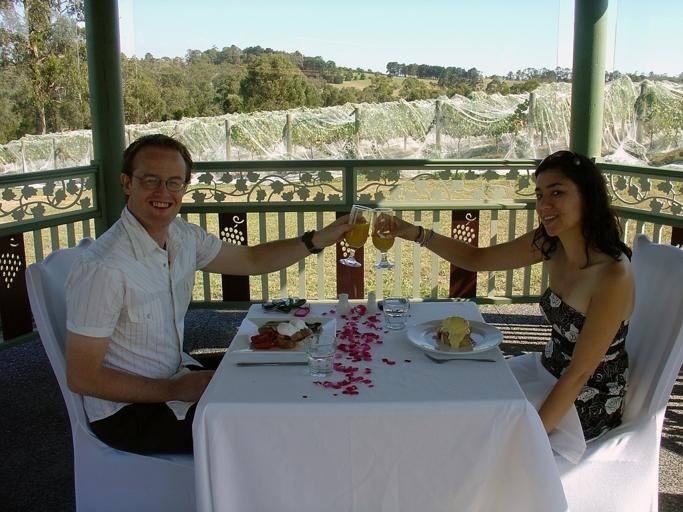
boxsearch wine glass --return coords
[371,208,397,269]
[340,204,372,268]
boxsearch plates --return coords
[231,317,337,356]
[407,318,505,355]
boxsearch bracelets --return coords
[301,229,324,256]
[413,225,435,248]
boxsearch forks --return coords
[424,353,496,364]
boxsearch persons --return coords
[375,148,636,446]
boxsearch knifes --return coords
[236,362,307,366]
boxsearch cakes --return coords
[431,316,472,350]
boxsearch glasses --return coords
[132,174,189,194]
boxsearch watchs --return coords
[61,132,366,458]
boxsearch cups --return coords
[382,296,410,329]
[303,334,336,378]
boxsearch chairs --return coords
[23,237,196,511]
[554,235,683,510]
